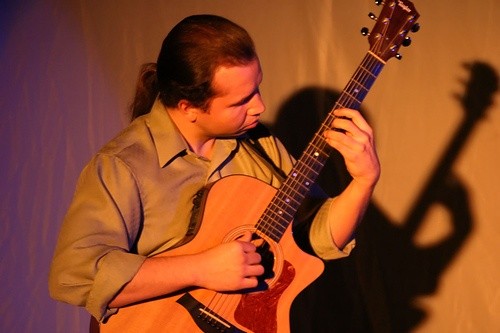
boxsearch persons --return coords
[47,16,382,333]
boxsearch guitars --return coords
[84,0,421,333]
